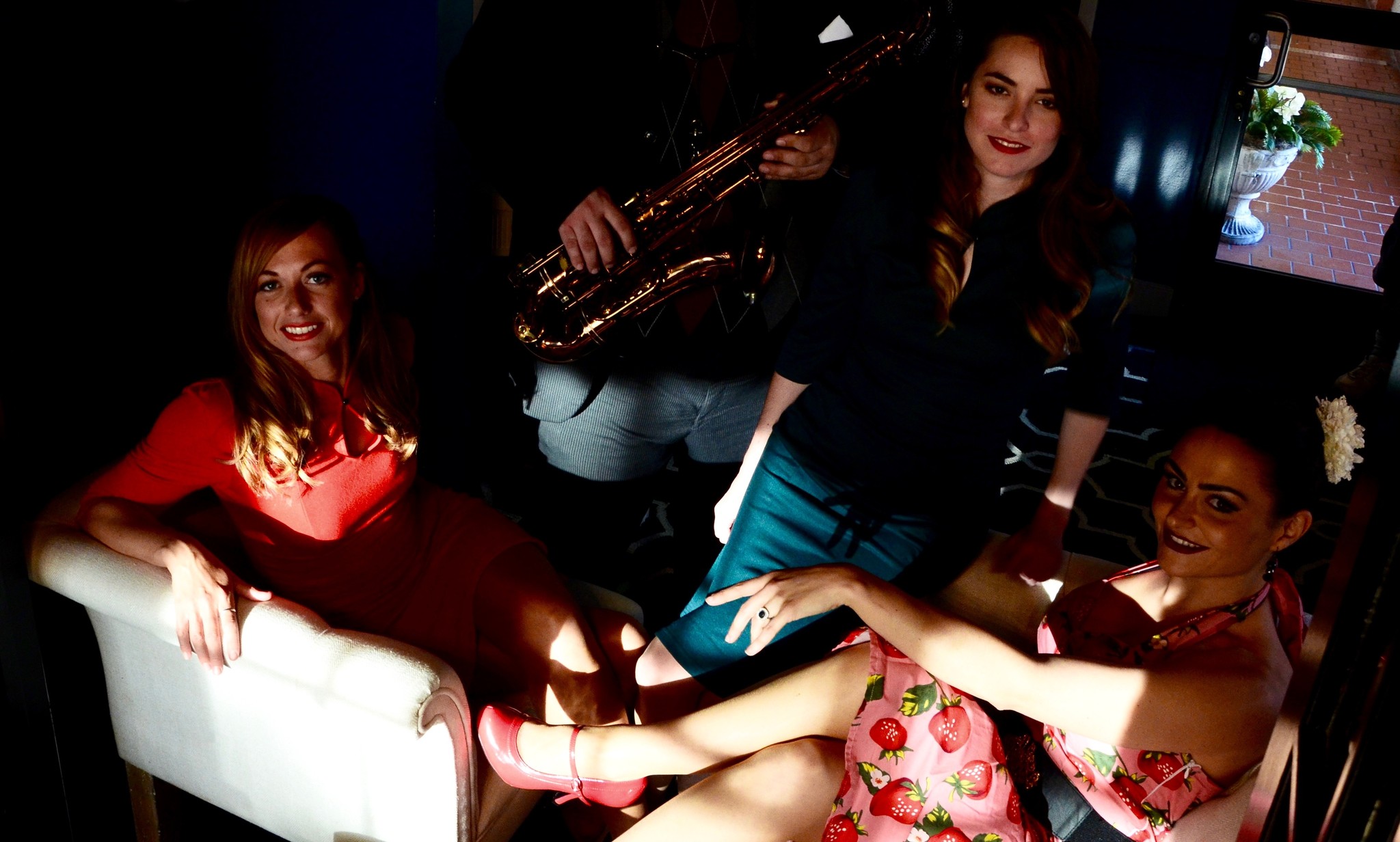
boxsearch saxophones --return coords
[508,3,946,359]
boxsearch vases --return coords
[1219,143,1301,243]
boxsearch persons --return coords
[476,397,1358,842]
[460,1,890,608]
[659,17,1140,686]
[74,203,673,842]
[1333,207,1399,393]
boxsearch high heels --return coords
[475,703,647,807]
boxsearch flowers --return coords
[1314,391,1369,486]
[1244,82,1344,171]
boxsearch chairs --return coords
[25,471,645,842]
